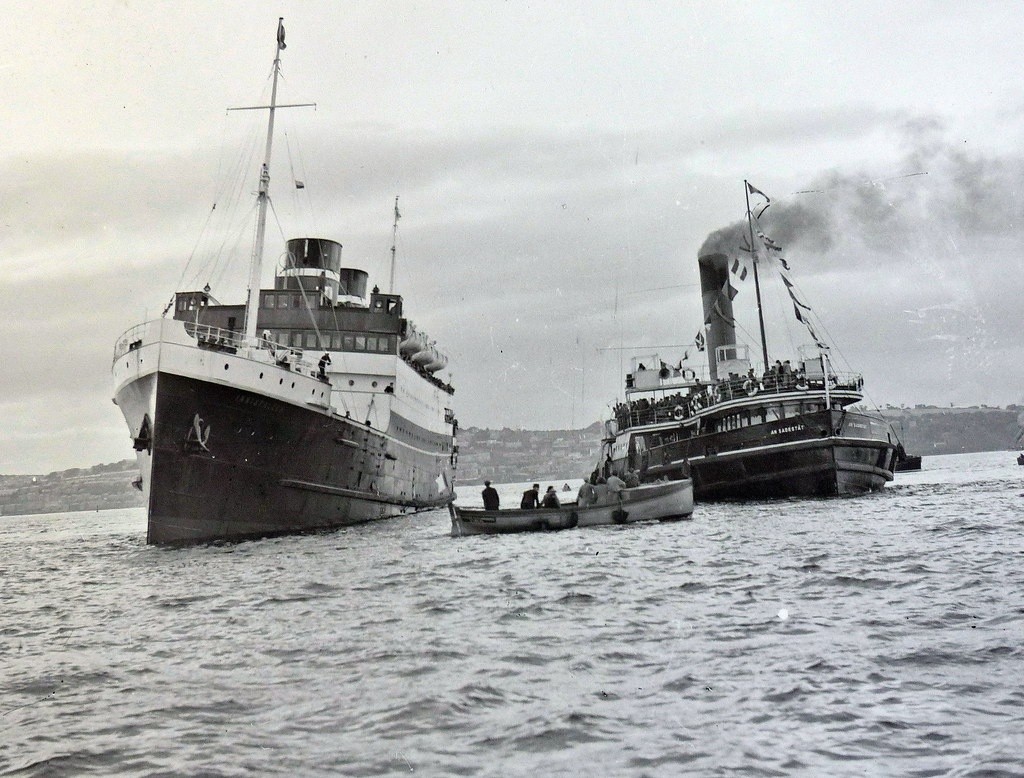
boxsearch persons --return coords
[540,486,561,509]
[576,475,598,504]
[607,470,626,500]
[625,466,638,488]
[482,481,500,511]
[613,360,806,433]
[596,476,609,498]
[521,484,539,510]
[318,352,331,375]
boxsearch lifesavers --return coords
[681,367,696,382]
[673,393,704,421]
[742,379,759,398]
[713,386,722,403]
[793,373,811,391]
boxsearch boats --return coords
[896,454,922,472]
[448,479,698,538]
[557,180,902,497]
[105,13,459,550]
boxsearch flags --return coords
[276,24,286,50]
[772,257,830,350]
[721,279,738,302]
[755,229,776,244]
[661,362,681,378]
[738,235,750,252]
[730,259,747,281]
[748,183,770,203]
[695,333,704,352]
[764,242,783,255]
[703,316,712,332]
[711,300,736,329]
[295,181,304,189]
